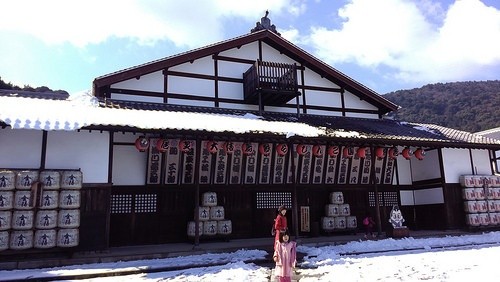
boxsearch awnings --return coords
[1,94,500,153]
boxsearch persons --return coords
[273,230,298,282]
[274,205,289,250]
[363,212,377,239]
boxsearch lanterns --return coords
[343,145,354,159]
[414,147,427,160]
[402,147,414,161]
[357,146,369,159]
[134,135,151,152]
[388,147,400,159]
[276,142,289,156]
[223,140,235,155]
[296,142,309,156]
[328,144,340,158]
[311,143,324,158]
[156,137,170,153]
[377,146,387,159]
[178,137,193,153]
[259,141,272,157]
[242,140,255,156]
[206,139,220,154]
